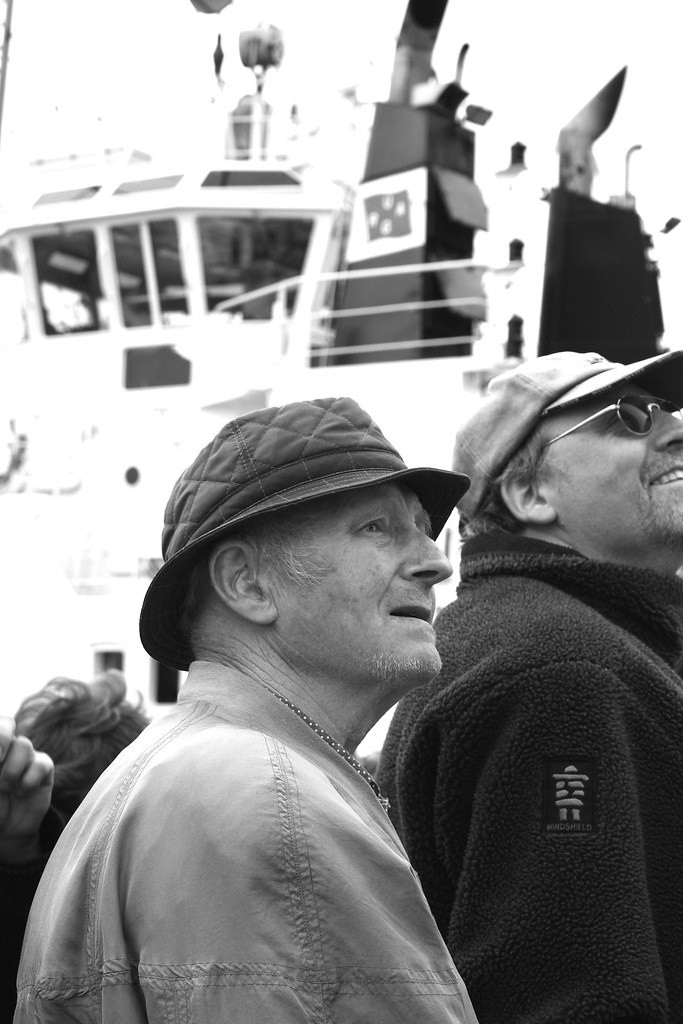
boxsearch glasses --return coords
[541,393,683,449]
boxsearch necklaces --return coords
[265,682,393,814]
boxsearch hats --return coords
[139,396,471,672]
[452,348,683,524]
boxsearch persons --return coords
[373,349,683,1023]
[0,395,480,1023]
[0,668,153,1023]
